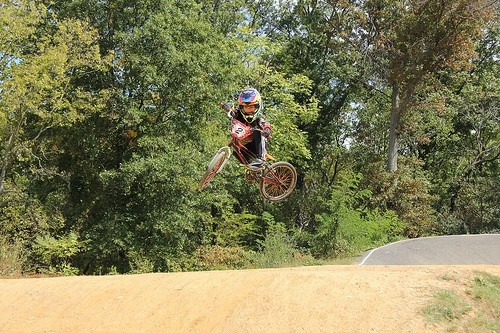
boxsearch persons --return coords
[223,87,272,176]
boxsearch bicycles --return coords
[198,103,297,202]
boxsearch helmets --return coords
[238,88,262,123]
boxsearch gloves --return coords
[263,128,271,138]
[222,102,233,112]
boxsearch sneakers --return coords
[248,161,265,171]
